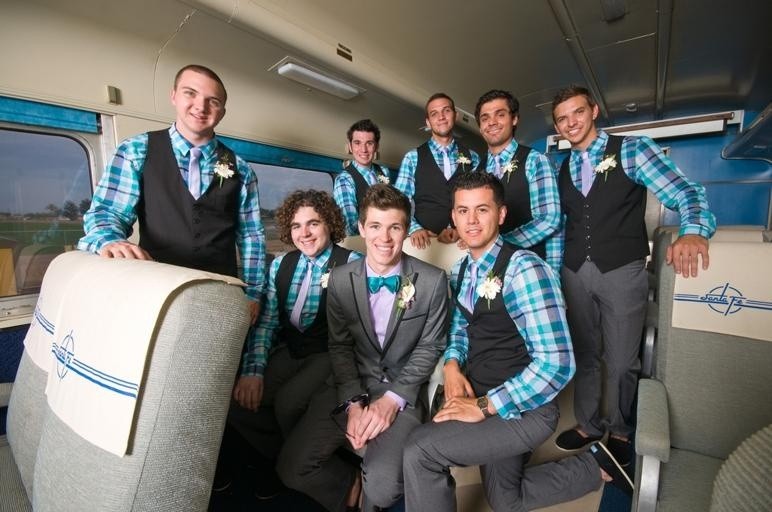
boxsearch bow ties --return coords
[365,275,400,294]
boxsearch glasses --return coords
[329,393,370,439]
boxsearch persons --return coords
[393,92,480,246]
[543,85,717,467]
[211,190,365,497]
[276,183,455,511]
[403,172,635,512]
[437,89,565,294]
[76,63,266,329]
[331,120,393,235]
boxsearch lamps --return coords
[267,54,368,102]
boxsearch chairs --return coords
[31,256,251,512]
[631,241,772,511]
[401,234,467,301]
[0,249,106,512]
[640,224,772,378]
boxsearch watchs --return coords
[478,396,492,418]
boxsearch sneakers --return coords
[254,468,281,499]
[608,430,634,467]
[212,473,232,491]
[554,422,607,451]
[588,441,635,492]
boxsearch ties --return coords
[580,151,592,198]
[369,172,379,185]
[441,148,452,181]
[290,261,314,333]
[188,146,202,201]
[466,263,478,315]
[493,155,501,177]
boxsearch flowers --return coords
[396,276,415,318]
[476,270,503,311]
[455,151,472,165]
[500,158,520,183]
[376,171,390,185]
[319,261,336,289]
[592,153,618,182]
[213,160,235,187]
